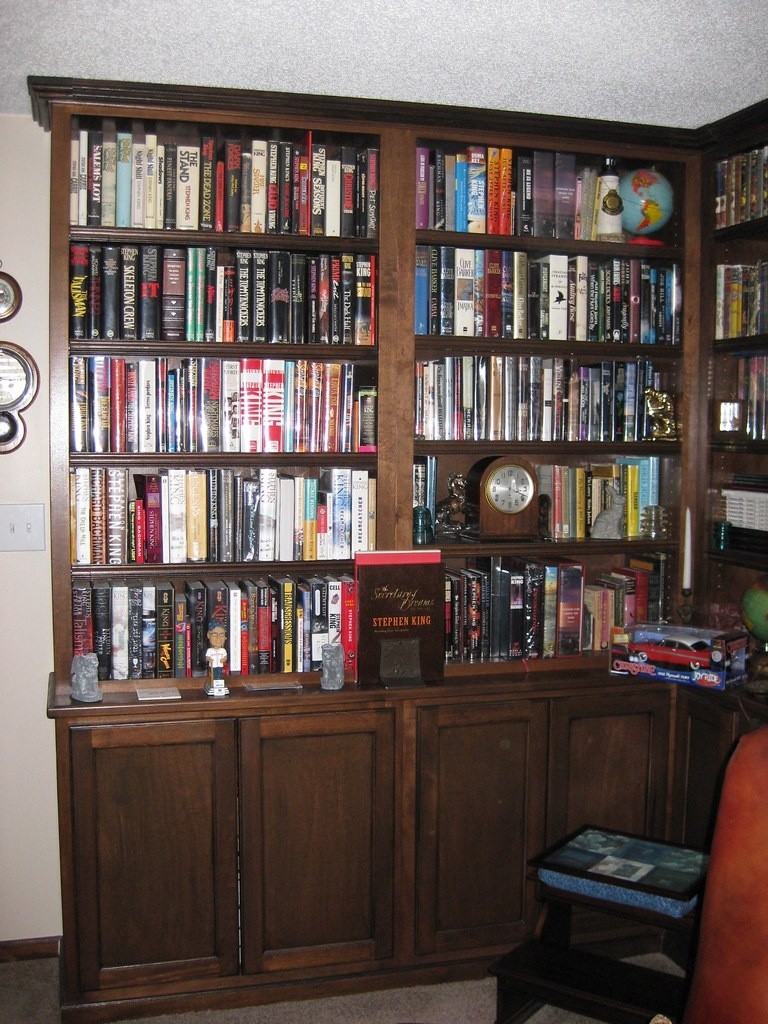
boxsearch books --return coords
[412,452,662,670]
[354,550,447,690]
[72,578,359,683]
[415,137,625,246]
[69,247,377,341]
[415,245,681,345]
[413,355,672,443]
[69,115,378,238]
[703,148,768,559]
[71,353,373,455]
[72,465,378,564]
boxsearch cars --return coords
[627,636,731,670]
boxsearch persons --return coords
[204,620,228,686]
[642,386,675,437]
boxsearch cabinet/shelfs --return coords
[24,75,768,1024]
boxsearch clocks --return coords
[461,456,540,542]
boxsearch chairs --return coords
[494,730,768,1024]
